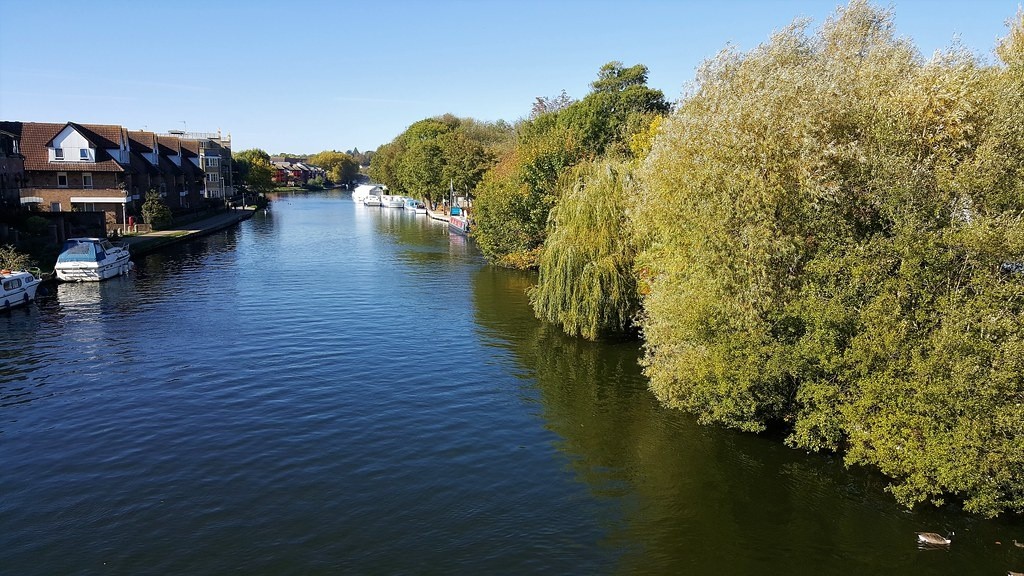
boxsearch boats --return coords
[0,266,43,311]
[364,194,427,214]
[55,237,135,281]
